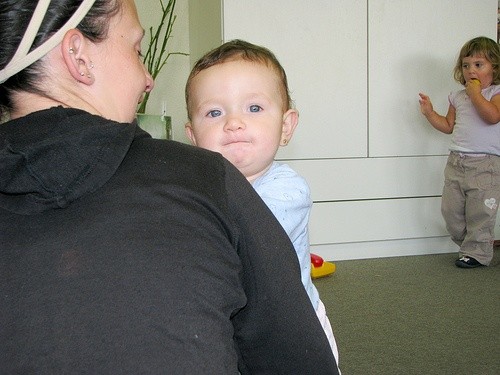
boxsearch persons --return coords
[0,0,341,375]
[416,35,500,269]
[185,37,339,375]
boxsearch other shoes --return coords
[455,256,481,268]
